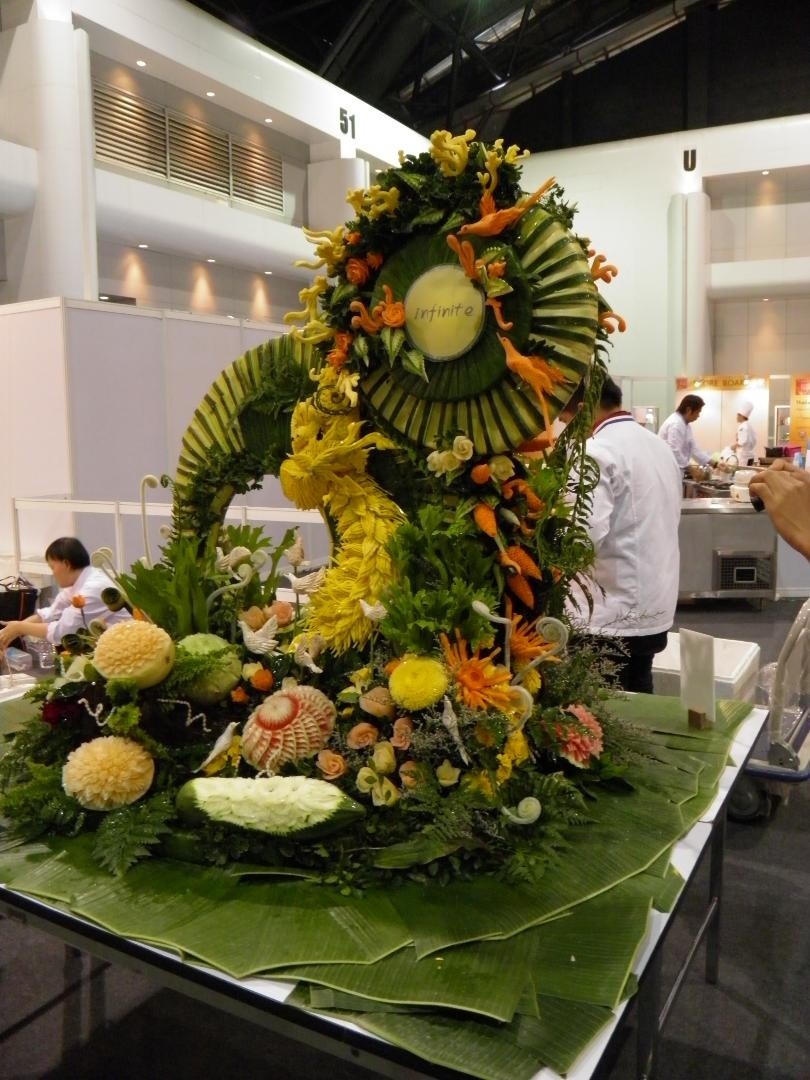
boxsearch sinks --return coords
[700,481,733,494]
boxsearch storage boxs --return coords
[652,631,760,705]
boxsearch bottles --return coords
[698,463,712,481]
[39,638,57,670]
[718,456,726,478]
[0,647,33,672]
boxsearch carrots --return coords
[327,192,627,711]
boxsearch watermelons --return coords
[239,684,337,774]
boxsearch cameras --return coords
[750,496,764,512]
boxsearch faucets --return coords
[725,455,739,469]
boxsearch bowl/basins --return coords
[764,445,801,457]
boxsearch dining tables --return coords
[0,682,768,1080]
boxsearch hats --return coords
[737,401,754,418]
[633,407,647,423]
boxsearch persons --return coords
[744,459,809,565]
[559,362,683,697]
[730,402,756,468]
[658,393,726,481]
[0,537,130,655]
